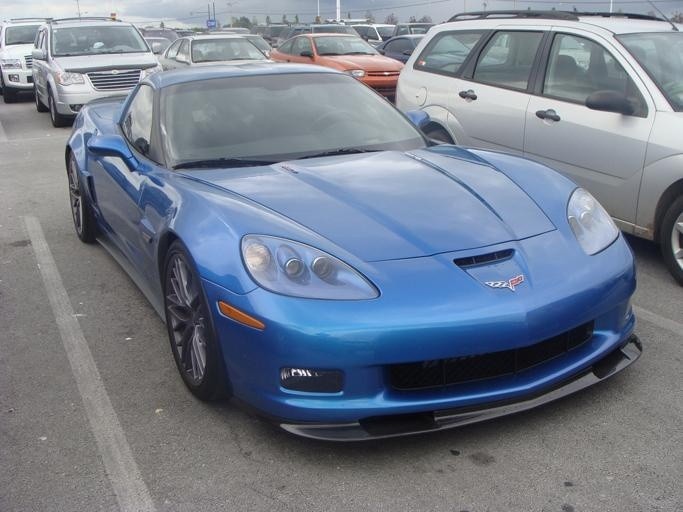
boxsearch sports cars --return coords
[63,62,644,444]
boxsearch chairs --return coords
[555,53,581,81]
[193,46,234,57]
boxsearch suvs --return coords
[397,10,683,286]
[1,17,59,103]
[33,18,162,127]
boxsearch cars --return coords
[140,20,434,102]
